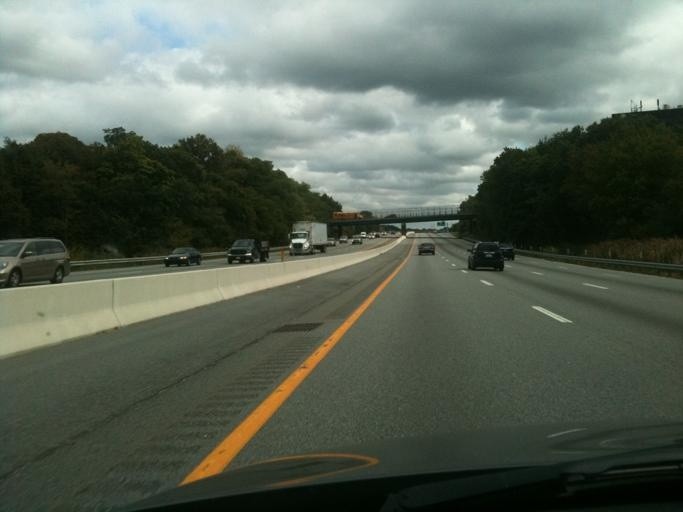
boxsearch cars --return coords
[327,231,387,246]
[467,240,515,271]
[417,243,435,255]
[164,246,202,267]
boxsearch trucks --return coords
[289,220,327,255]
[333,212,363,220]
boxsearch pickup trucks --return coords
[227,239,270,263]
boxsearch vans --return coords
[0,237,71,287]
